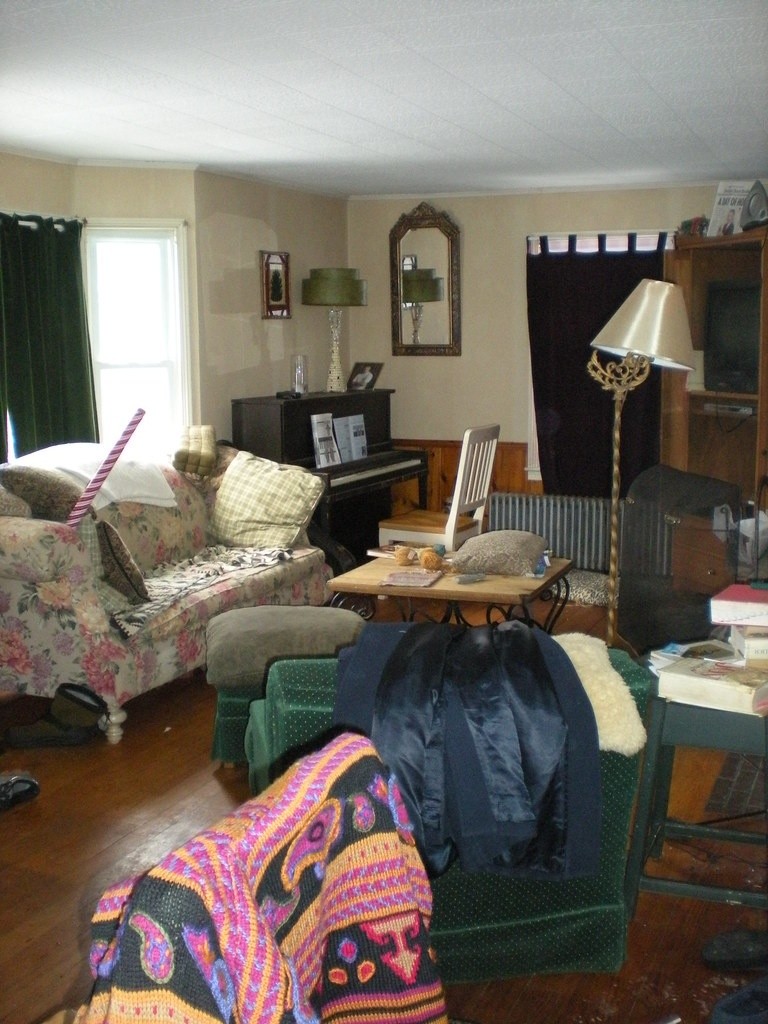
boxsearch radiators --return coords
[487,492,669,578]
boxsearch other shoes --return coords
[702,931,768,969]
[711,973,768,1024]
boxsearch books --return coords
[648,625,768,717]
[711,583,768,628]
[367,544,419,562]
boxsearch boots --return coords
[3,683,107,747]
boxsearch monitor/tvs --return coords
[702,278,761,395]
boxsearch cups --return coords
[291,354,308,396]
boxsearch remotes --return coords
[456,572,485,584]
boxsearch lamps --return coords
[301,267,368,392]
[586,279,697,650]
[402,269,444,344]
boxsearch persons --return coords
[350,364,374,390]
[717,209,735,235]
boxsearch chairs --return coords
[243,649,651,981]
[379,423,501,553]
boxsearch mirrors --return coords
[389,202,462,356]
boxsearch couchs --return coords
[0,447,333,744]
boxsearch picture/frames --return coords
[260,250,292,320]
[348,363,384,390]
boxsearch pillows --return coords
[0,464,96,521]
[205,606,365,685]
[92,519,152,603]
[207,452,325,549]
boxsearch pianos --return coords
[229,388,431,534]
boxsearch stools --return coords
[212,688,266,770]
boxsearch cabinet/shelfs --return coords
[671,228,768,596]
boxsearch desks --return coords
[327,554,572,635]
[626,643,768,925]
